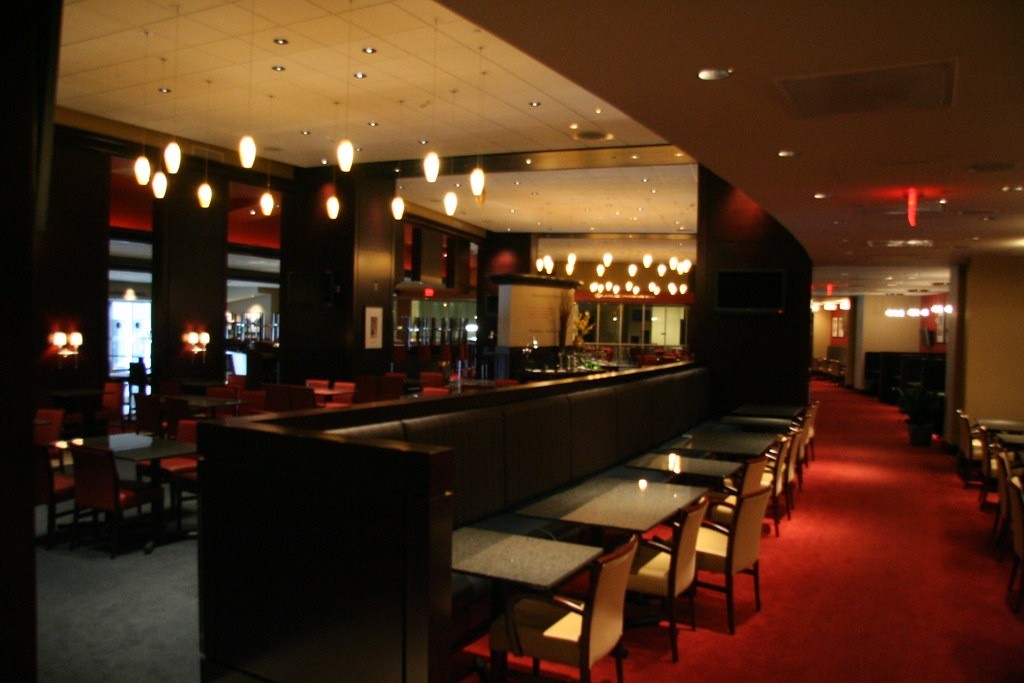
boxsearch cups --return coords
[447,359,489,393]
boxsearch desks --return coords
[976,418,1024,432]
[521,363,607,380]
[661,416,793,457]
[451,527,604,683]
[513,450,744,532]
[995,433,1024,451]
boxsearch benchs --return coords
[197,360,713,683]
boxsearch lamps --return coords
[134,0,486,220]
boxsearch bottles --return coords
[409,316,470,343]
[225,310,280,354]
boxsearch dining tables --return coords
[70,432,198,553]
[47,388,115,428]
[172,396,241,419]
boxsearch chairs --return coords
[956,407,1024,609]
[668,401,820,634]
[488,534,639,683]
[30,340,521,559]
[812,358,844,382]
[626,495,709,663]
